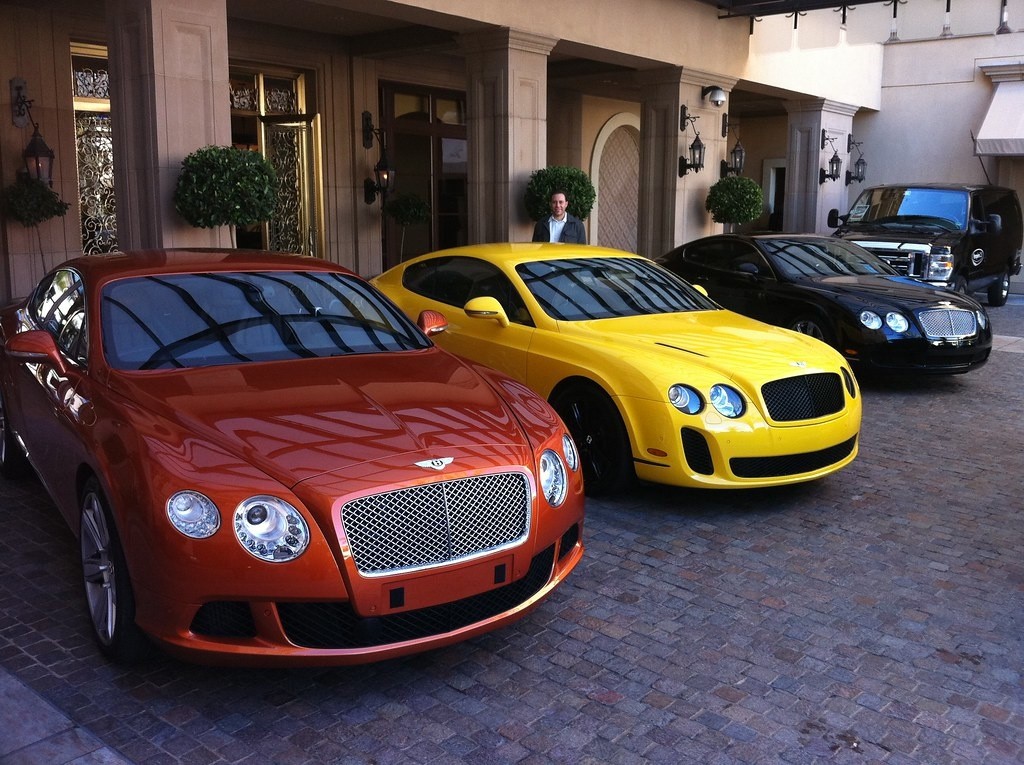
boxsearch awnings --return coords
[974,81,1024,156]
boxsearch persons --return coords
[532,191,586,243]
[768,199,783,231]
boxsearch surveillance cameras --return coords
[709,88,726,109]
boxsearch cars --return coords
[0,245,590,673]
[369,241,863,499]
[655,231,993,391]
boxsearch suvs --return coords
[824,178,1023,305]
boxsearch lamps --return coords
[361,110,397,207]
[679,104,706,177]
[720,112,746,177]
[883,0,1015,45]
[845,133,868,186]
[9,77,55,189]
[833,5,858,53]
[819,129,843,184]
[702,85,728,110]
